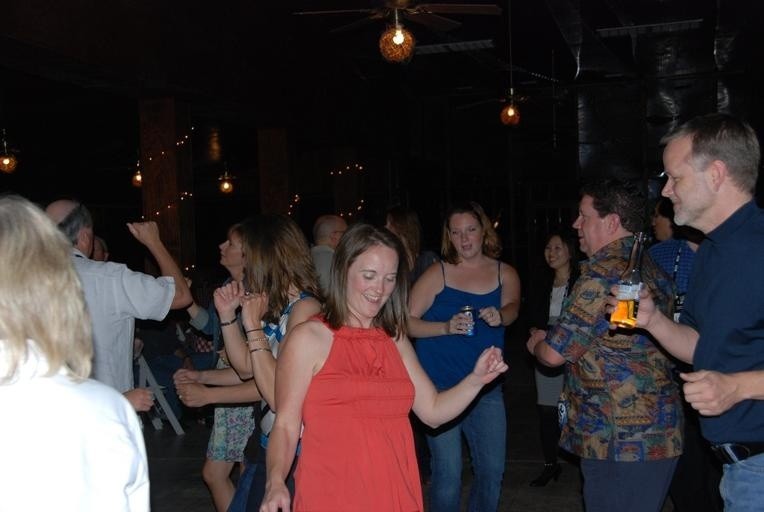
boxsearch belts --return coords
[708,441,764,466]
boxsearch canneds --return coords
[462,305,476,337]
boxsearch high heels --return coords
[529,466,561,488]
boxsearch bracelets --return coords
[220,318,238,327]
[249,348,272,354]
[245,328,264,334]
[246,338,269,344]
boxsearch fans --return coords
[294,1,497,33]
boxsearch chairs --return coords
[130,351,185,436]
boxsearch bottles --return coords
[609,230,650,329]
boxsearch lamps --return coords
[497,13,521,127]
[131,160,143,187]
[218,166,235,193]
[0,128,16,172]
[378,2,415,65]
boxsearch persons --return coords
[643,193,705,328]
[527,230,580,487]
[528,174,685,508]
[45,200,349,510]
[262,221,509,510]
[376,213,436,294]
[401,203,521,508]
[0,195,150,510]
[607,115,762,509]
